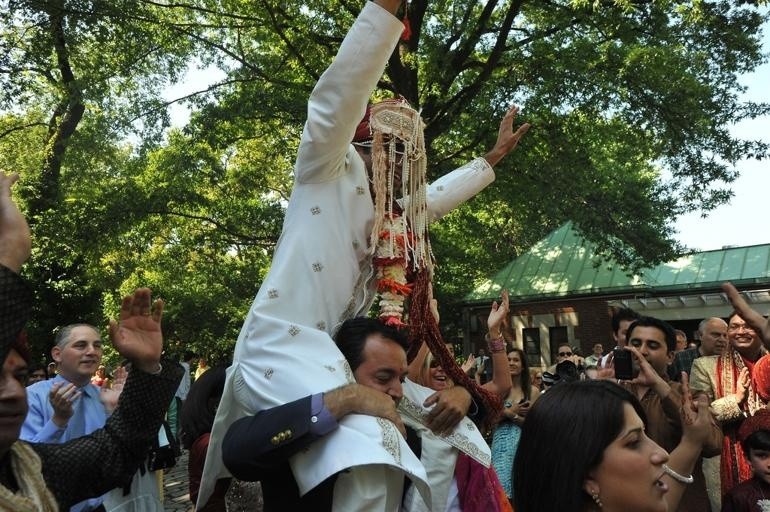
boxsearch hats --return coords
[348,95,428,160]
[735,408,770,443]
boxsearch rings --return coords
[140,311,152,318]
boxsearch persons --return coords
[192,0,531,512]
[221,312,479,512]
[19,321,129,511]
[26,356,50,388]
[173,343,191,452]
[0,168,185,512]
[93,361,113,388]
[194,356,212,384]
[48,361,57,380]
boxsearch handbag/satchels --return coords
[147,419,182,472]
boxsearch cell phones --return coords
[613,349,634,380]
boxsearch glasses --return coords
[558,351,573,357]
[429,358,441,369]
